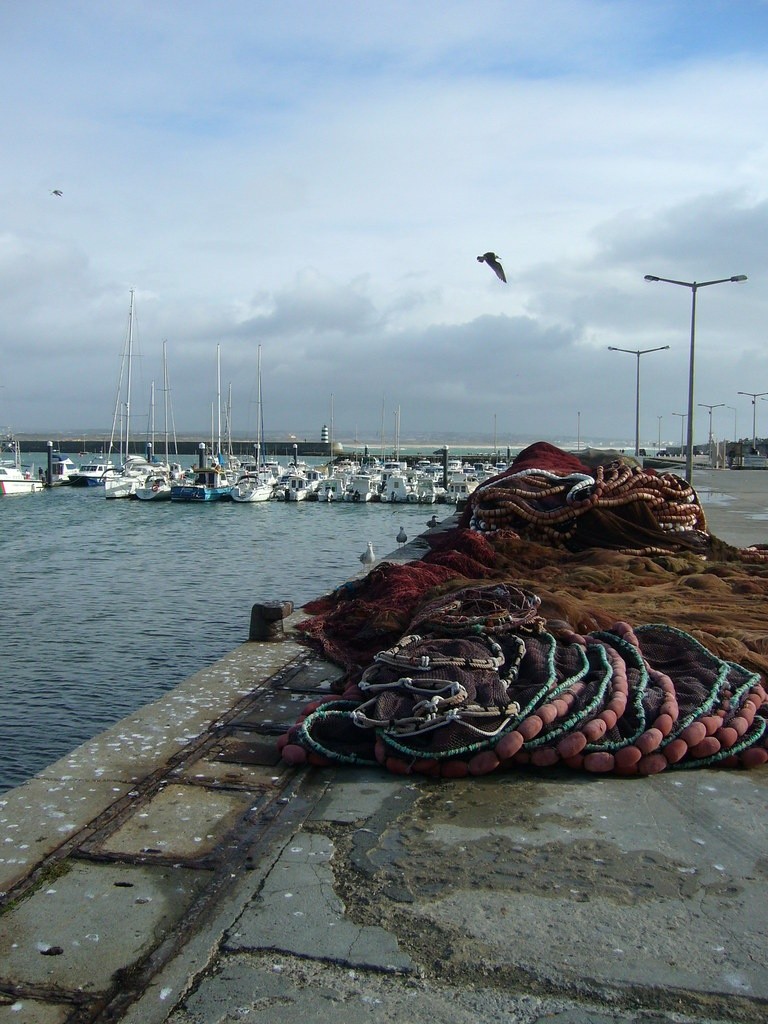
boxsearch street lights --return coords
[697,402,726,461]
[671,413,690,457]
[608,344,670,456]
[656,414,664,455]
[736,390,768,455]
[645,274,748,483]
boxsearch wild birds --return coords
[396,526,408,548]
[427,515,439,529]
[359,542,376,571]
[51,190,64,198]
[477,252,507,282]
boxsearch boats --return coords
[0,439,45,494]
[44,290,516,503]
